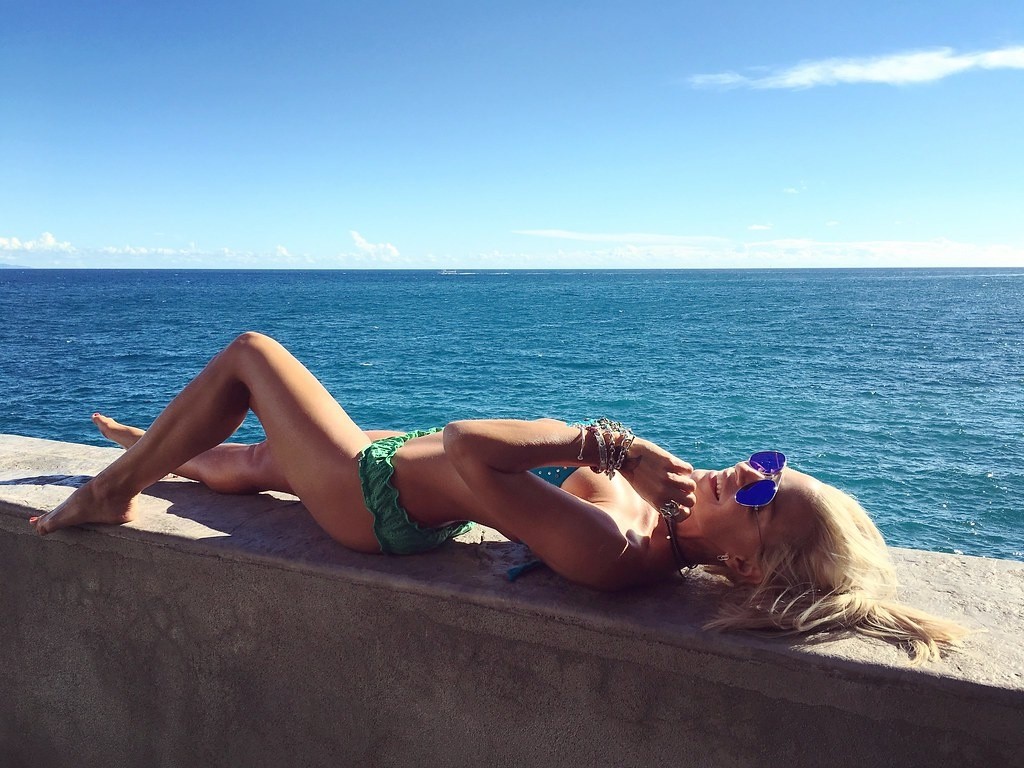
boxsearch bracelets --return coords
[574,417,635,482]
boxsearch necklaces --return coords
[665,519,698,578]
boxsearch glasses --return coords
[735,451,786,554]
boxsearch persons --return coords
[28,330,968,665]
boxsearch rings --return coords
[658,502,677,517]
